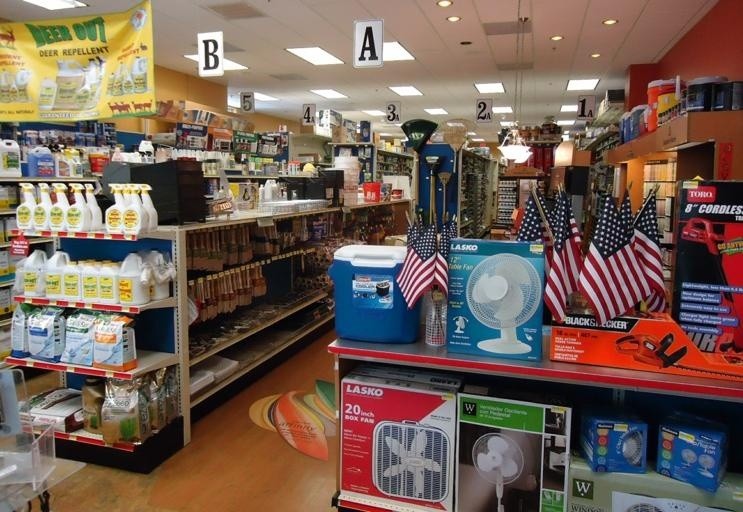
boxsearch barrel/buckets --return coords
[619,75,742,144]
[363,182,381,203]
[0,54,147,111]
[264,179,280,202]
[1,131,171,304]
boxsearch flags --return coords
[396,208,458,310]
[514,181,667,326]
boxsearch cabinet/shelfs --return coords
[548,111,740,319]
[1,121,409,474]
[328,337,743,512]
[489,176,549,229]
[290,135,499,244]
